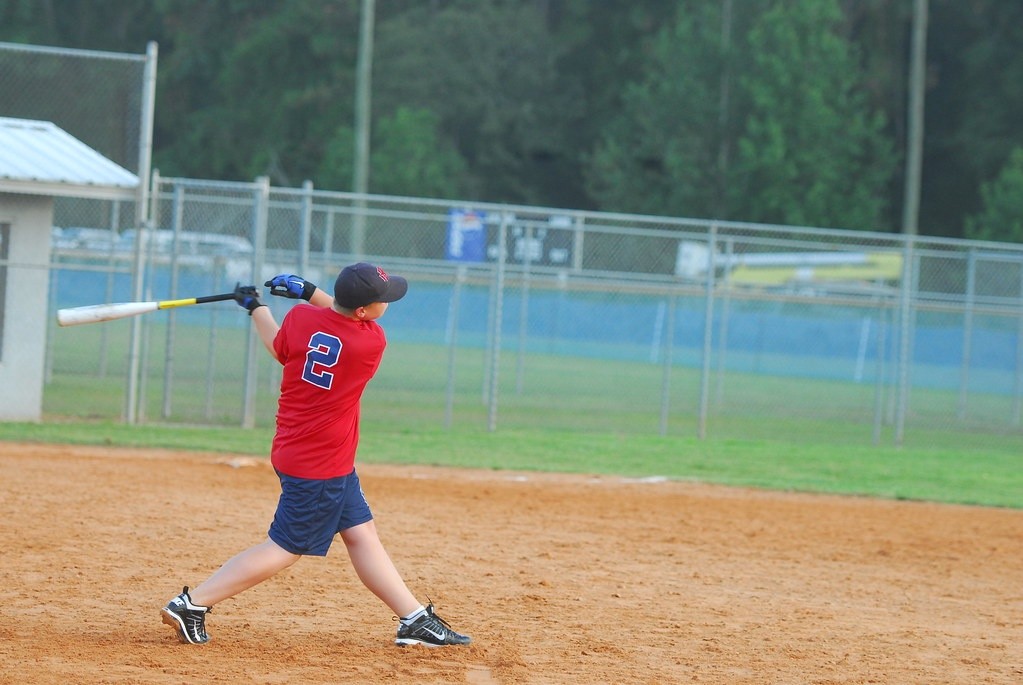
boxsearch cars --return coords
[42,224,251,269]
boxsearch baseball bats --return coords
[56,288,262,327]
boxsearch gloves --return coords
[264,274,315,302]
[234,282,267,313]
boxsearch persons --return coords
[161,263,470,650]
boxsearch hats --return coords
[334,262,407,308]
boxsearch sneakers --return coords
[160,586,210,645]
[393,604,472,649]
